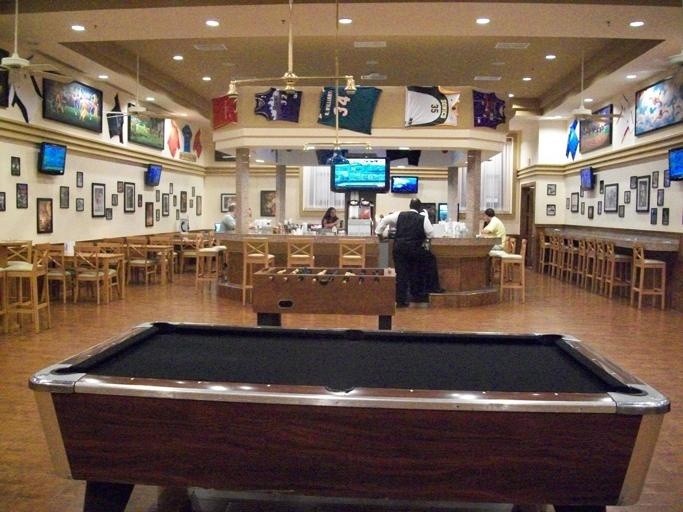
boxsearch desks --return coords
[29,321,665,511]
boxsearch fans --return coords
[537,50,622,123]
[0,0,74,84]
[106,56,184,119]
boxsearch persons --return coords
[483,213,491,229]
[321,206,343,229]
[375,198,445,306]
[481,208,506,250]
[217,203,236,281]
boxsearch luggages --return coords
[409,238,441,297]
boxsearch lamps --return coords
[298,62,374,151]
[226,0,356,100]
[326,142,349,165]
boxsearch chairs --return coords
[339,238,365,269]
[539,231,667,311]
[0,232,227,334]
[243,237,275,306]
[287,237,316,268]
[488,235,527,304]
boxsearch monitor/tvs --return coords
[146,164,162,186]
[331,157,390,193]
[391,176,418,193]
[668,147,683,181]
[438,203,459,222]
[581,166,593,190]
[39,142,66,175]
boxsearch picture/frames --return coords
[567,170,671,225]
[128,103,164,150]
[634,76,683,136]
[1,158,202,233]
[547,184,556,195]
[547,204,556,216]
[579,103,613,154]
[261,191,276,216]
[42,71,103,133]
[221,193,237,212]
